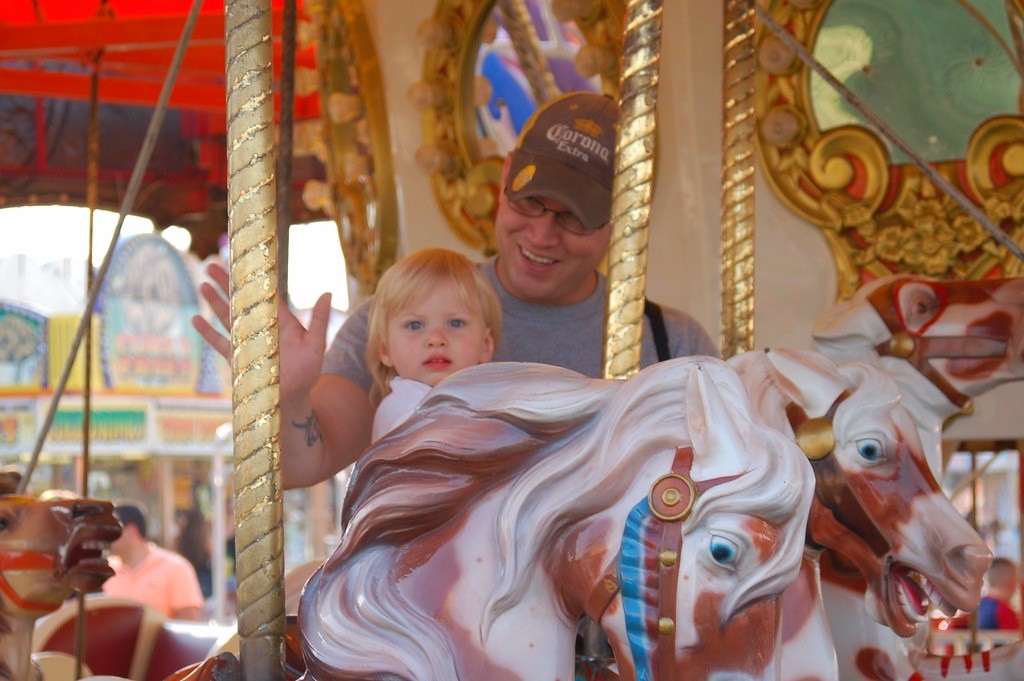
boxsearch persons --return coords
[225,565,238,616]
[101,505,213,621]
[365,248,503,447]
[191,92,719,492]
[978,557,1020,631]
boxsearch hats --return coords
[506,93,621,228]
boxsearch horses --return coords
[300,354,815,681]
[0,472,124,681]
[762,346,994,681]
[811,273,1024,488]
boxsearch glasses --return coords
[503,187,609,236]
